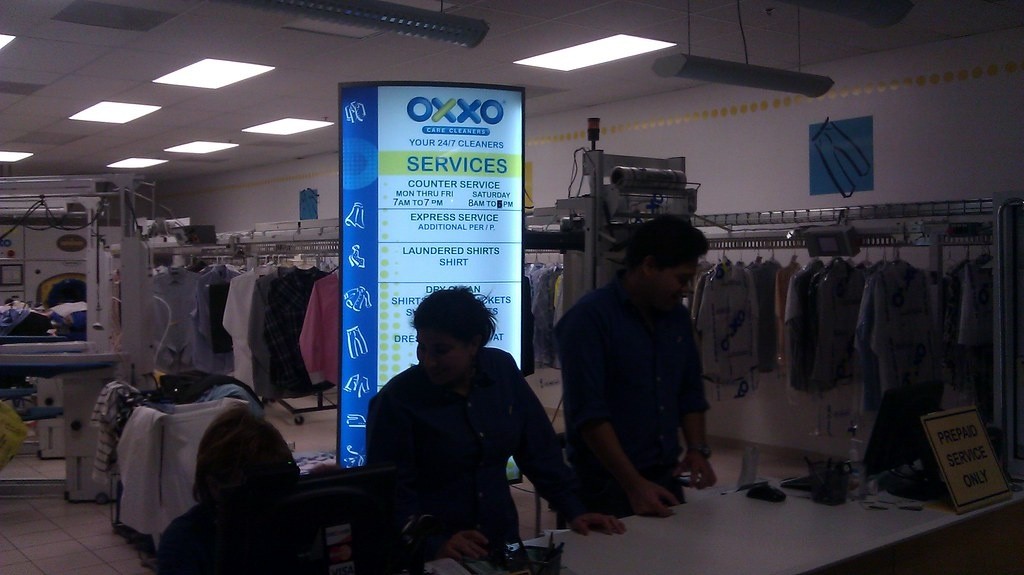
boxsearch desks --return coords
[403,461,1024,575]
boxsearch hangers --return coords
[533,229,995,267]
[212,236,341,277]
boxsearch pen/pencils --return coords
[535,532,565,575]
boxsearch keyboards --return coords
[780,470,813,493]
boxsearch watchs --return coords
[686,444,711,457]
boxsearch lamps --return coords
[208,0,492,48]
[651,0,835,99]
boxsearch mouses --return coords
[747,485,786,502]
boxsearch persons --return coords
[155,406,327,575]
[554,215,717,518]
[366,289,627,561]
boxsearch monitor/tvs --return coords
[860,377,941,501]
